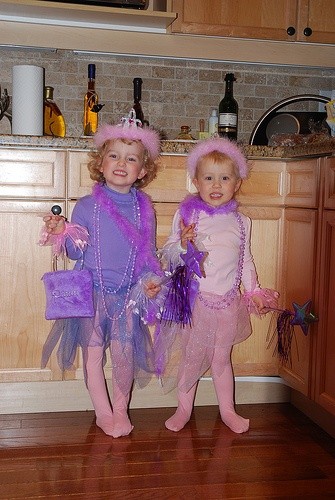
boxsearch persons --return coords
[160,137,279,433]
[37,126,165,438]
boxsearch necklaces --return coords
[86,195,141,321]
[189,210,245,311]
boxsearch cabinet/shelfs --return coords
[0,0,177,29]
[171,0,335,45]
[278,157,335,416]
[0,146,77,381]
[64,149,281,379]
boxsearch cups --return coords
[11,65,44,137]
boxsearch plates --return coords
[266,113,300,141]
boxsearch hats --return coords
[95,107,159,157]
[188,138,248,179]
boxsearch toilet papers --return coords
[10,64,45,138]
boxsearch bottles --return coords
[131,78,144,125]
[208,110,218,138]
[83,64,99,136]
[43,86,66,138]
[218,73,238,143]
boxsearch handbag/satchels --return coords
[41,232,95,320]
[161,256,200,323]
